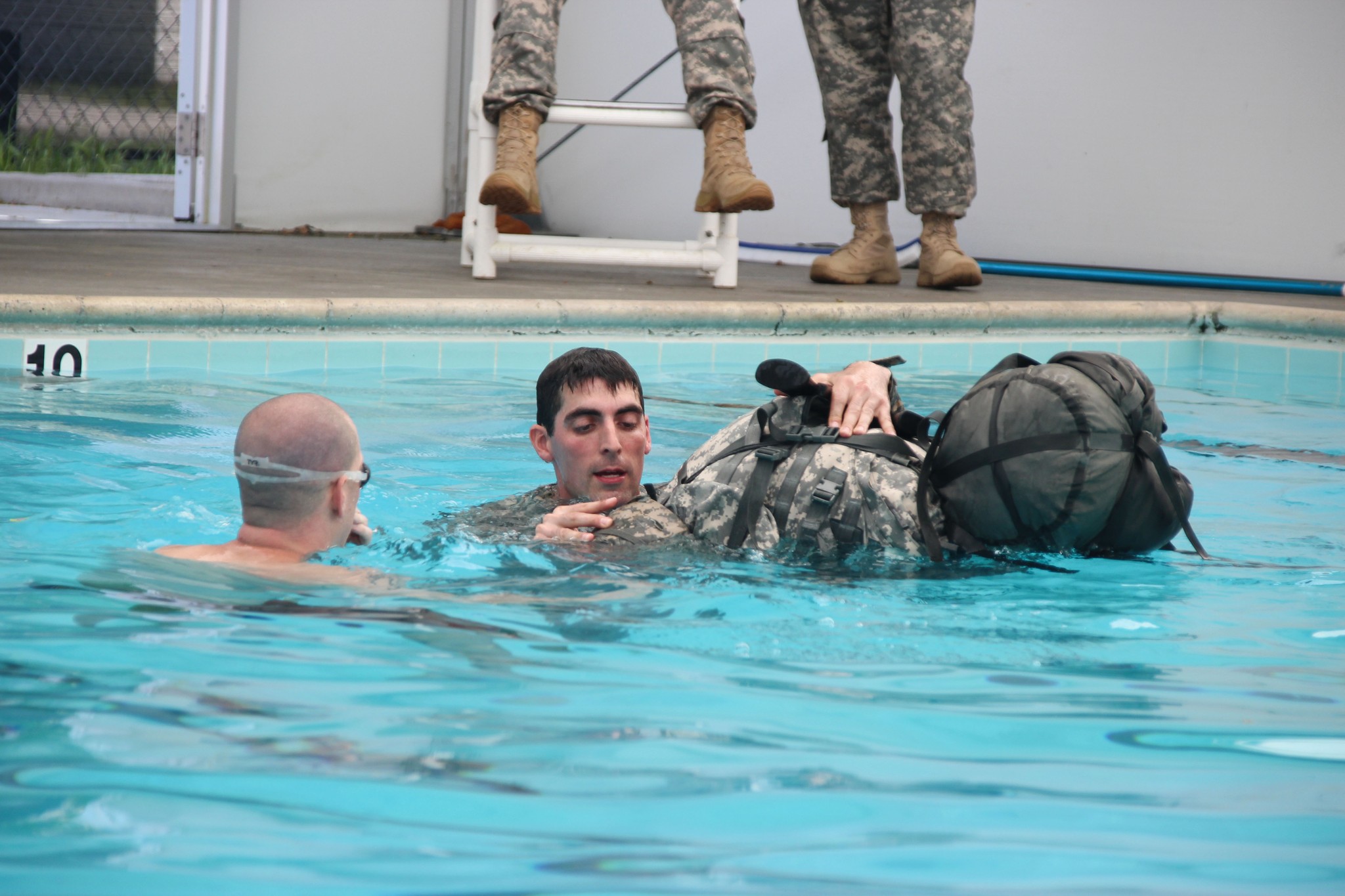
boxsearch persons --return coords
[153,392,373,569]
[796,0,983,287]
[447,346,896,555]
[482,0,774,225]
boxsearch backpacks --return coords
[654,350,1213,568]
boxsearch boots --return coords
[694,103,774,213]
[811,201,900,284]
[916,212,982,288]
[480,102,542,216]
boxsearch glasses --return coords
[232,453,371,487]
